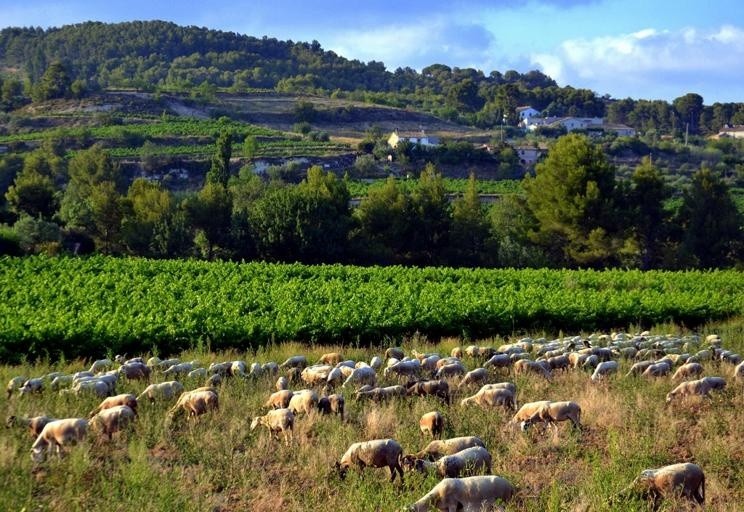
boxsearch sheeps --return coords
[607,461,708,511]
[5,330,743,485]
[408,474,515,511]
